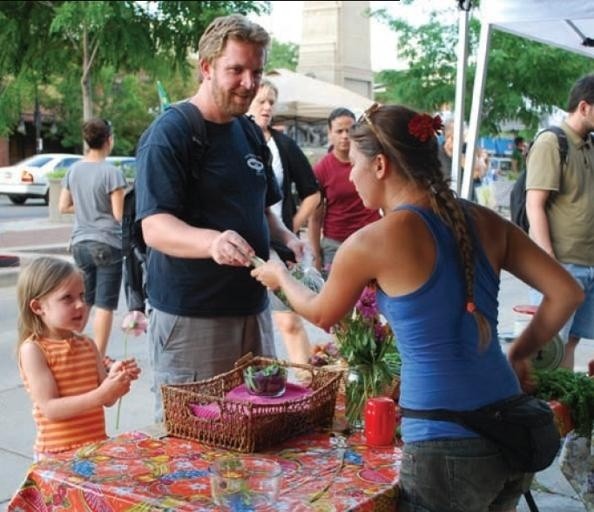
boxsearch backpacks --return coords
[509,127,569,234]
[121,103,270,296]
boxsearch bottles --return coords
[364,396,396,449]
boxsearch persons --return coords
[134,13,307,423]
[119,181,147,316]
[244,76,323,374]
[14,256,141,464]
[249,101,587,512]
[435,114,489,202]
[57,117,129,370]
[307,106,385,330]
[524,72,594,378]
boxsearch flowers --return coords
[340,293,393,359]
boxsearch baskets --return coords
[159,348,346,456]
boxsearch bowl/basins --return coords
[243,365,288,397]
[210,457,280,511]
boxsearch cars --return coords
[103,155,136,169]
[0,153,82,205]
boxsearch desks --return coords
[5,341,576,512]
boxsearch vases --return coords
[346,361,394,436]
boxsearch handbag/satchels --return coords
[398,394,561,474]
